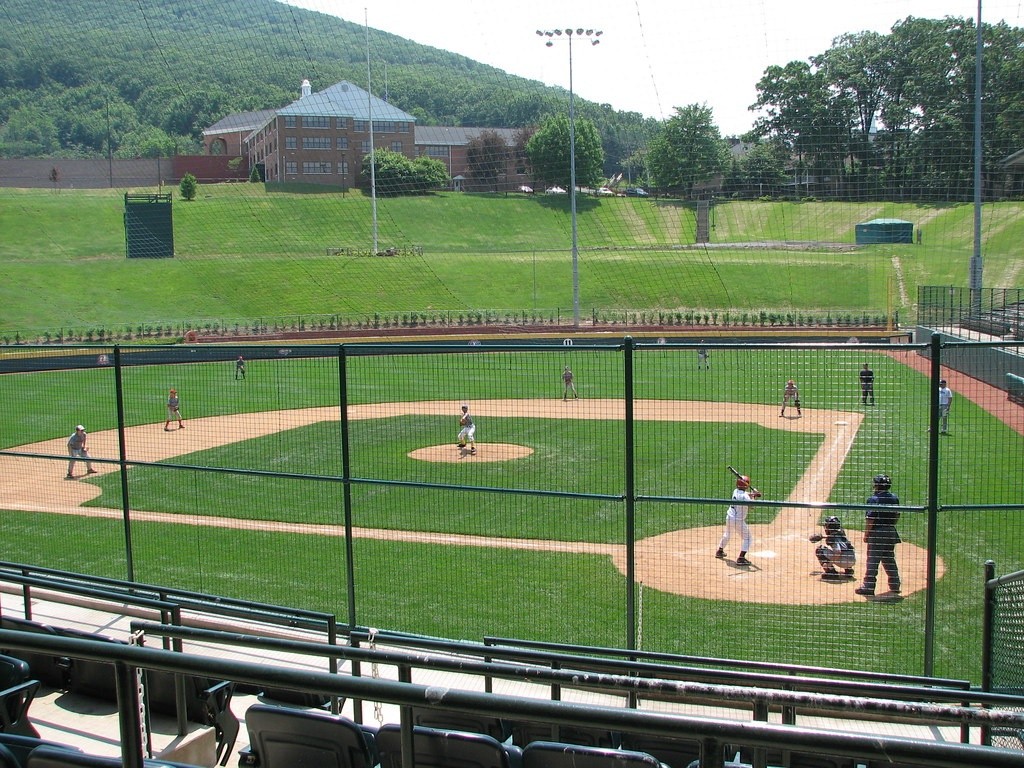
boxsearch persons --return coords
[928,379,952,434]
[779,380,801,417]
[67,425,98,478]
[458,405,475,452]
[808,516,856,580]
[696,340,709,370]
[164,390,185,431]
[860,363,874,403]
[855,474,902,595]
[561,366,578,400]
[235,357,245,380]
[715,475,761,565]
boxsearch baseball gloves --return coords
[795,400,800,406]
[82,447,89,452]
[459,419,467,425]
[809,533,822,543]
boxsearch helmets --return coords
[737,475,750,489]
[939,379,946,385]
[824,516,840,524]
[462,405,468,411]
[873,474,891,489]
[788,380,794,384]
[76,424,86,431]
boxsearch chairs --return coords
[0,612,843,768]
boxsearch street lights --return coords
[534,26,605,329]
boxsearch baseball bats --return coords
[727,465,753,490]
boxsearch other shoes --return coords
[471,446,475,451]
[888,586,899,591]
[164,426,169,431]
[715,551,727,557]
[822,571,837,578]
[855,588,874,596]
[87,468,98,473]
[459,443,466,447]
[844,569,854,577]
[179,425,185,428]
[737,557,751,565]
[67,473,74,477]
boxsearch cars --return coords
[623,188,648,196]
[592,188,616,197]
[516,186,534,194]
[545,186,566,195]
